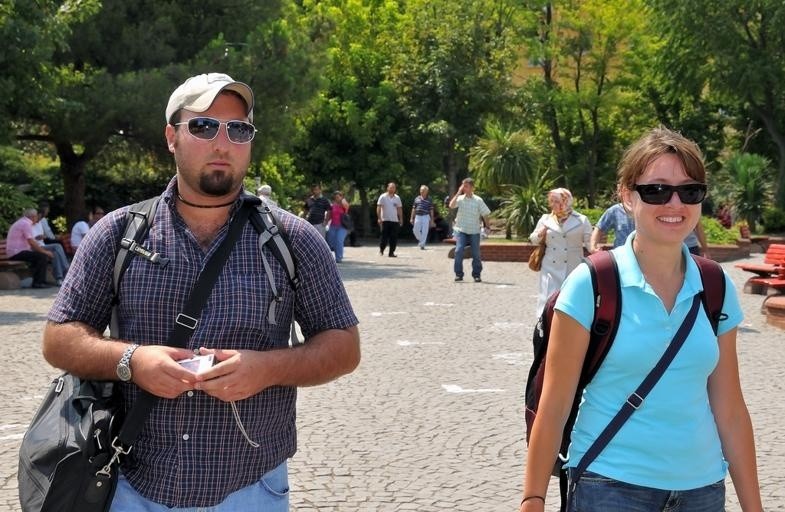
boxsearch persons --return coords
[377,184,404,258]
[517,131,768,512]
[588,200,637,254]
[31,206,72,285]
[325,191,354,264]
[258,185,278,207]
[5,209,53,287]
[684,219,712,262]
[300,183,331,238]
[528,187,597,323]
[409,185,437,250]
[89,207,104,229]
[449,178,493,283]
[41,71,360,512]
[69,208,94,255]
[40,200,67,254]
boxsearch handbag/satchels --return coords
[528,225,549,272]
[340,210,354,230]
[17,195,263,511]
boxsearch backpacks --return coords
[524,250,729,476]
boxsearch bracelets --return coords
[520,496,545,507]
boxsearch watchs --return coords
[115,343,140,382]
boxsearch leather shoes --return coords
[454,276,463,281]
[473,275,481,282]
[32,282,51,289]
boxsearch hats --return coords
[256,185,272,193]
[165,72,255,125]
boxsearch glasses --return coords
[627,183,707,205]
[95,213,105,215]
[173,116,260,145]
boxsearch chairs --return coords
[734,243,785,278]
[746,274,785,297]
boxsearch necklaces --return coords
[173,191,240,209]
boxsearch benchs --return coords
[740,224,769,254]
[0,234,78,279]
